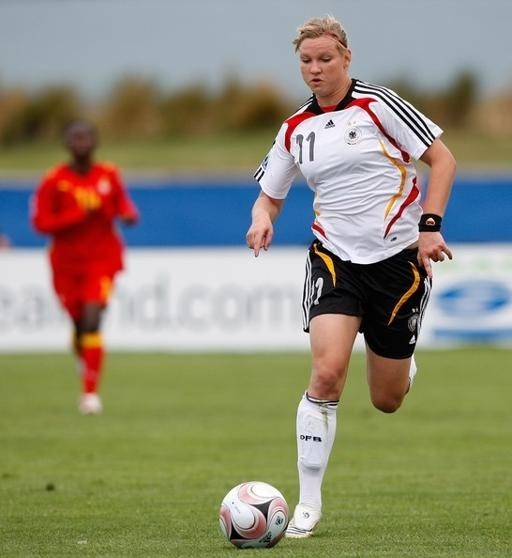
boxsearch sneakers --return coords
[284,503,322,540]
[79,393,101,415]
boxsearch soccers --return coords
[219,481,289,549]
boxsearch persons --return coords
[244,12,458,542]
[31,119,141,417]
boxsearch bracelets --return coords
[417,211,443,234]
